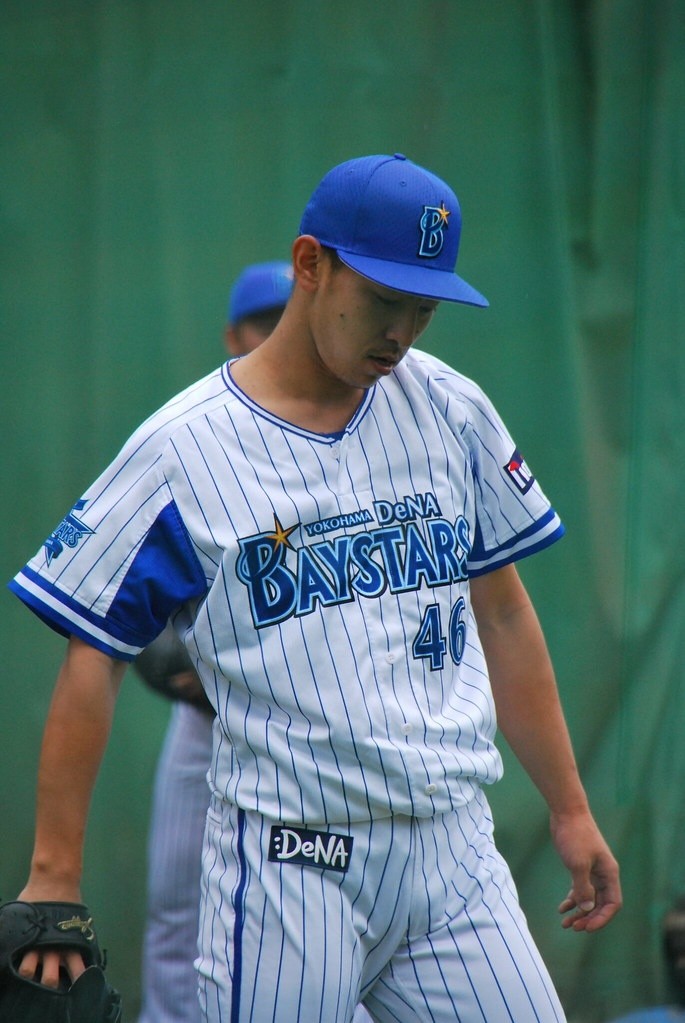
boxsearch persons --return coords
[0,155,624,1022]
[132,263,294,1022]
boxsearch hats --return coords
[228,261,298,328]
[299,153,489,307]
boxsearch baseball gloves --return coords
[1,898,126,1022]
